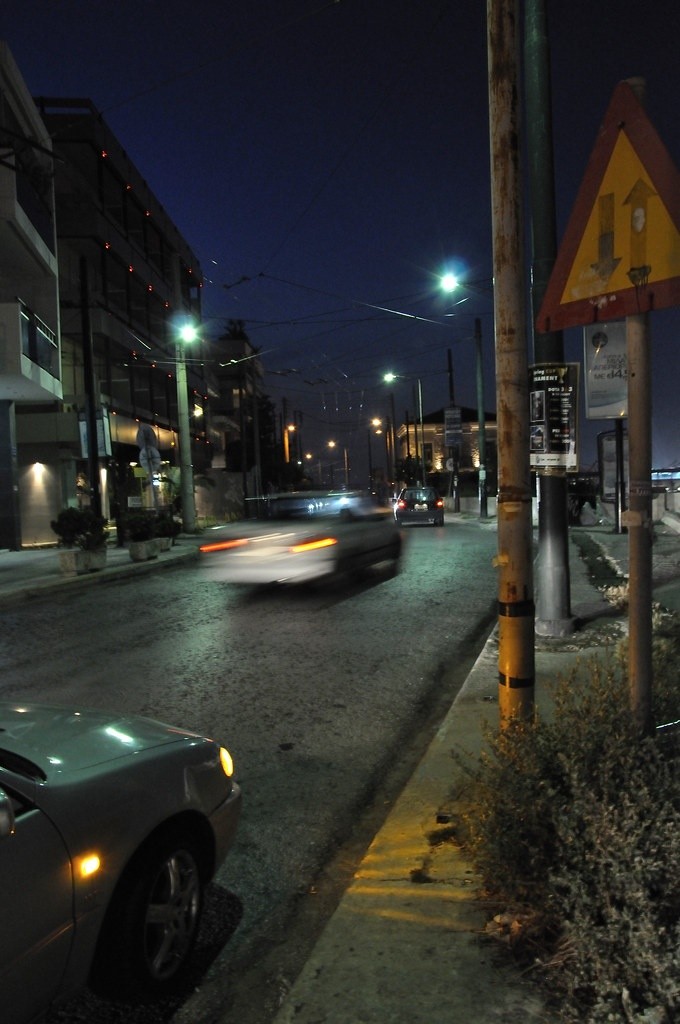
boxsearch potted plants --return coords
[122,505,175,561]
[49,506,109,573]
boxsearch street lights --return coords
[385,371,426,485]
[437,272,487,518]
[174,318,205,531]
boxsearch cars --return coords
[0,698,243,1024]
[198,491,400,589]
[393,487,444,526]
